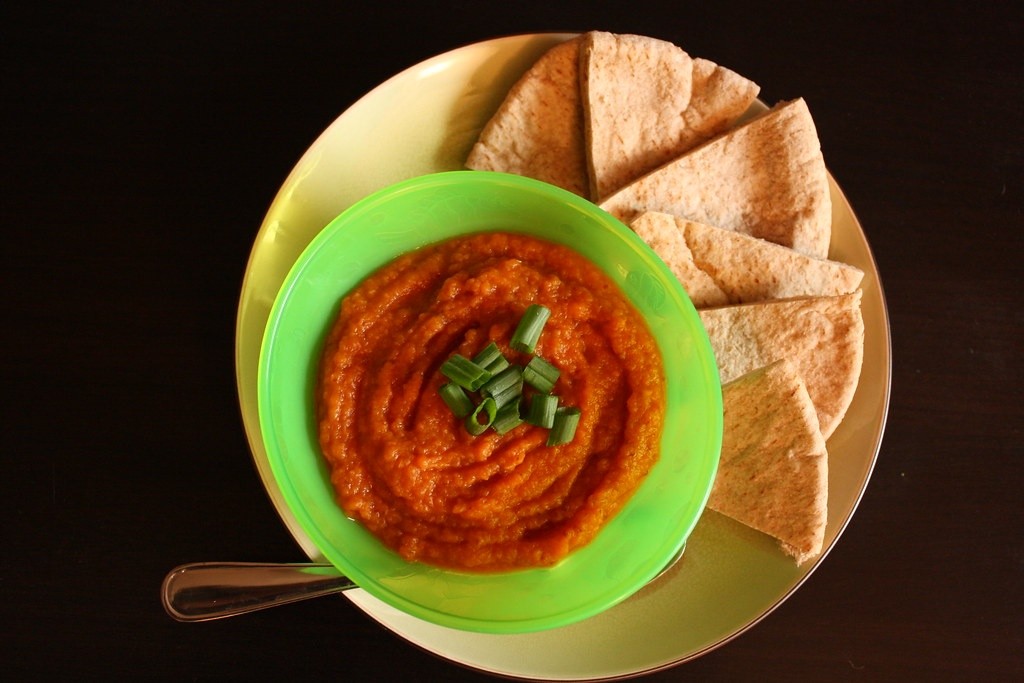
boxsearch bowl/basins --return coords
[255,170,725,632]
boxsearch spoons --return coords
[165,523,683,624]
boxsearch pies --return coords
[467,32,866,564]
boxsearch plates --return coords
[233,32,893,683]
